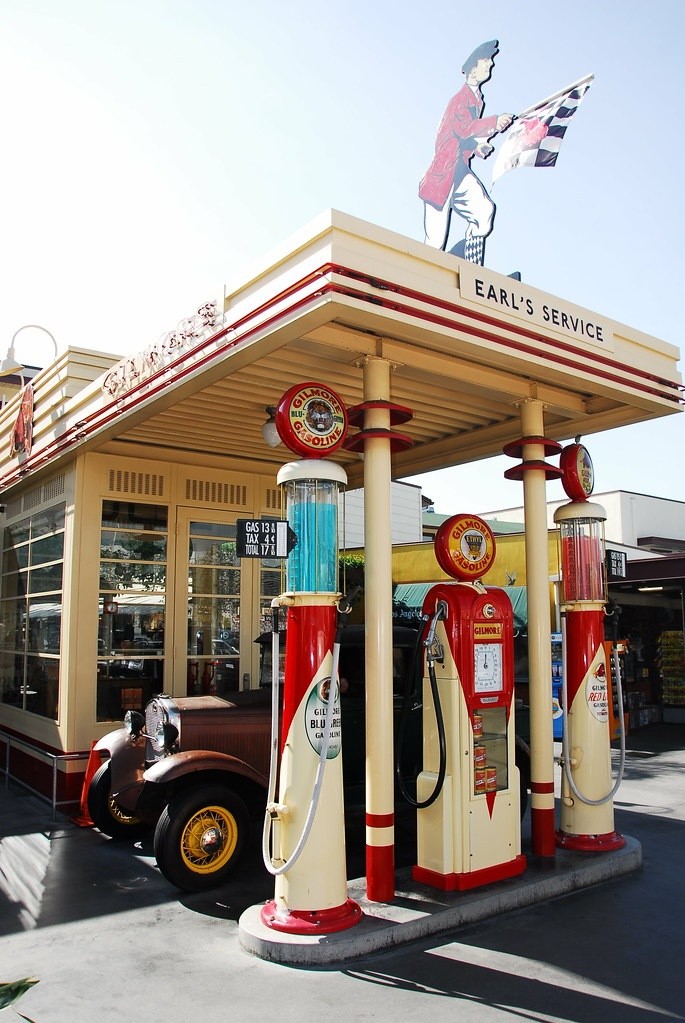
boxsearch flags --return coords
[493,83,591,181]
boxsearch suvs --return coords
[86,619,549,895]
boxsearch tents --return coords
[23,593,166,619]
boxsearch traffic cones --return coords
[67,739,102,828]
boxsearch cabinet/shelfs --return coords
[470,707,509,795]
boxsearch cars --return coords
[189,624,232,641]
[202,639,239,661]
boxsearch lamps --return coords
[261,407,282,448]
[0,325,58,376]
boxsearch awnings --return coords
[393,583,527,626]
